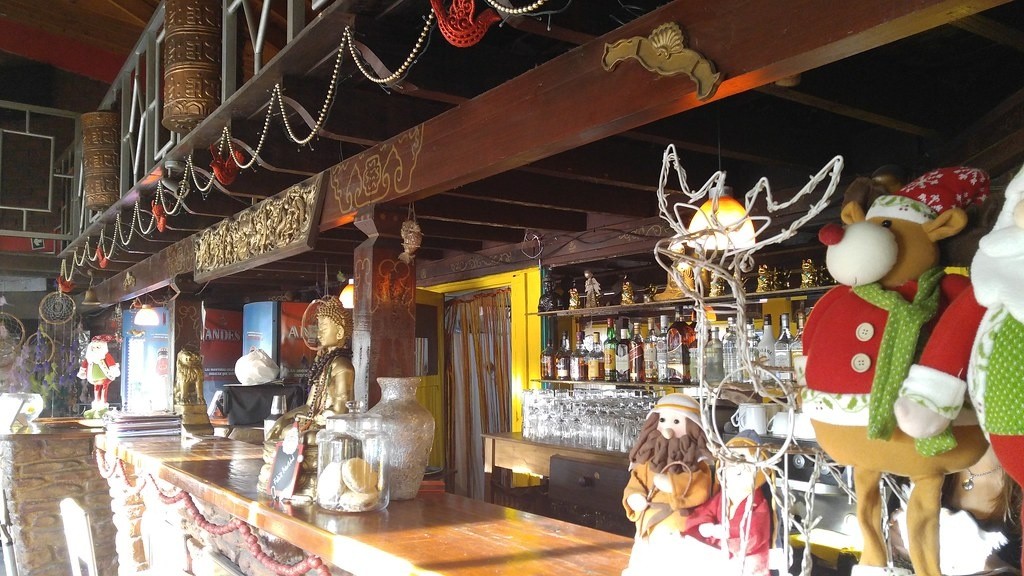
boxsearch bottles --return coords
[706,313,807,384]
[264,392,288,439]
[542,306,698,383]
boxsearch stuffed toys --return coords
[793,168,989,576]
[893,165,1024,489]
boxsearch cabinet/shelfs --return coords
[479,280,839,542]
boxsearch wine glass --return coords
[522,387,660,452]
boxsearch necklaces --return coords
[302,348,353,430]
[962,466,1002,491]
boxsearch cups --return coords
[730,400,817,439]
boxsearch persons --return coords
[621,392,773,576]
[538,276,557,311]
[76,333,121,407]
[266,296,355,503]
